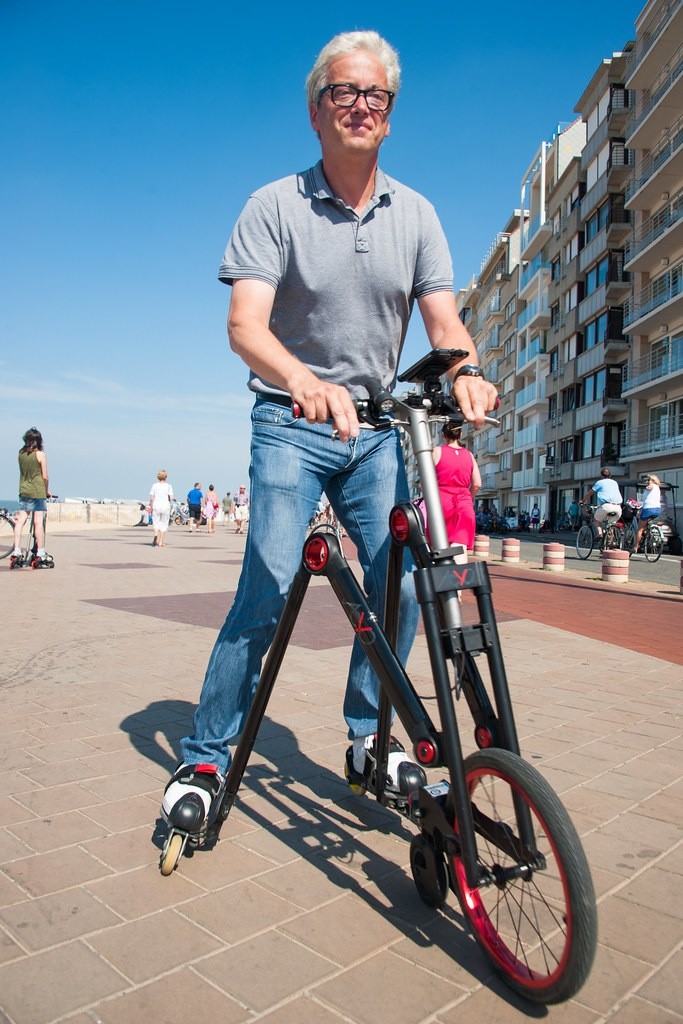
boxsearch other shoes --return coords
[13,554,25,561]
[153,536,157,545]
[206,530,215,533]
[196,529,202,532]
[593,534,603,542]
[629,547,637,554]
[190,529,192,532]
[159,544,165,547]
[235,527,243,534]
[36,553,53,561]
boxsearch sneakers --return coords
[163,763,224,833]
[353,735,415,792]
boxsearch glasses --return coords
[240,488,245,489]
[319,84,394,111]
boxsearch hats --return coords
[572,500,575,502]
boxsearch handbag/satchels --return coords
[213,502,218,508]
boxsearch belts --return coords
[236,504,244,506]
[256,392,368,423]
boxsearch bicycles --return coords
[576,503,625,561]
[621,503,664,563]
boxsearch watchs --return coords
[453,365,485,382]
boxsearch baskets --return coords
[579,506,592,521]
[622,506,636,522]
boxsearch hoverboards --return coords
[9,494,58,570]
[156,377,599,1010]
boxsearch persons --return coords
[162,30,502,841]
[577,468,623,546]
[132,483,250,534]
[530,503,541,532]
[430,422,483,597]
[10,427,54,561]
[629,474,661,553]
[568,500,578,533]
[149,470,174,547]
